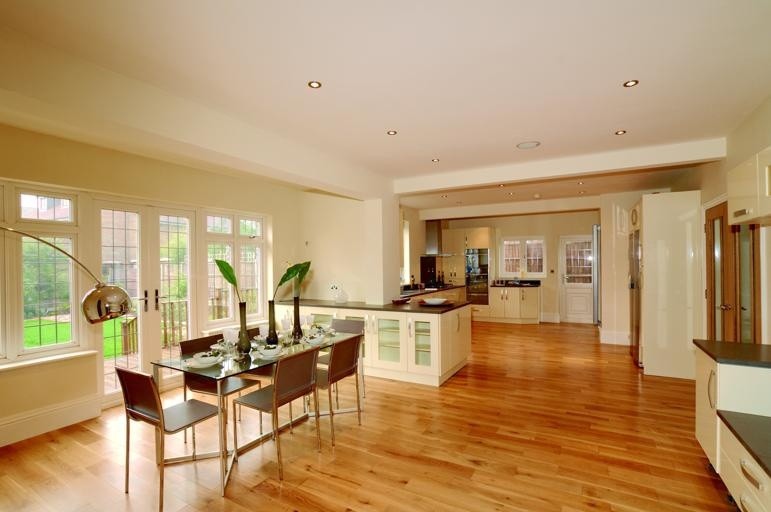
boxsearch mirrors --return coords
[466,248,488,305]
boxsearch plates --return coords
[417,300,454,306]
[182,356,228,370]
[209,323,336,361]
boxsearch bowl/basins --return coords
[194,351,222,363]
[424,298,447,305]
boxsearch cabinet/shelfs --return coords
[274,299,472,387]
[491,285,541,325]
[465,227,495,248]
[471,306,489,322]
[715,409,771,512]
[405,287,465,301]
[728,144,771,227]
[628,189,708,383]
[691,338,771,473]
[442,228,466,286]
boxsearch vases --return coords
[266,299,278,345]
[291,296,303,338]
[237,301,251,355]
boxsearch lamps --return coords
[1,226,134,325]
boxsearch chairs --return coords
[306,332,364,447]
[179,332,264,460]
[232,345,322,481]
[314,319,366,409]
[238,327,260,339]
[112,366,224,511]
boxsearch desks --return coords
[152,326,364,497]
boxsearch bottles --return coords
[426,268,445,285]
